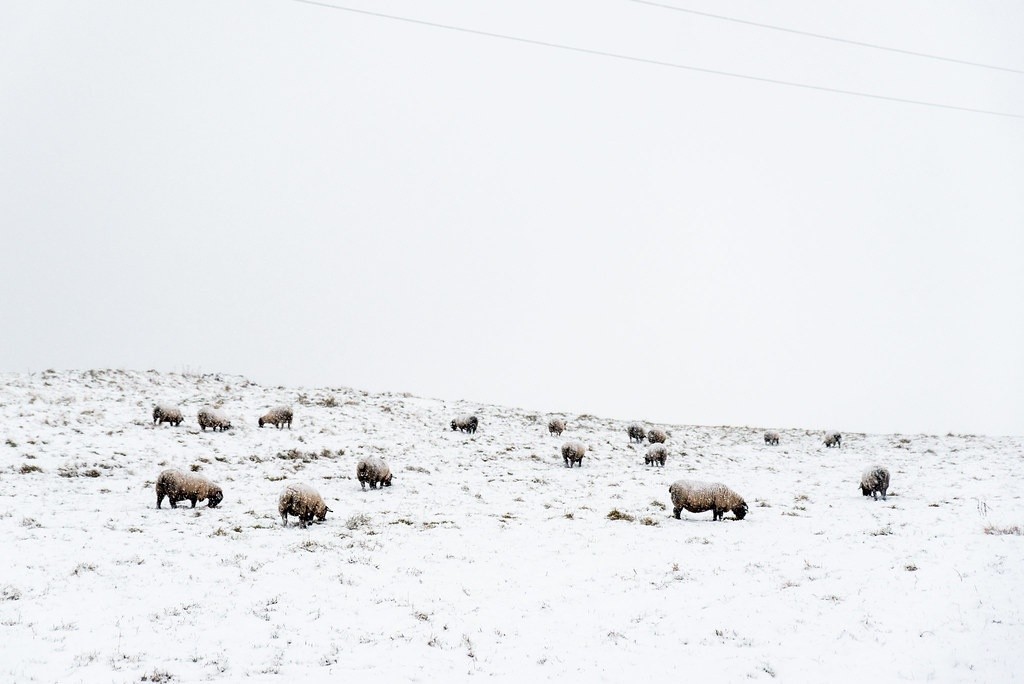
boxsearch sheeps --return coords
[627,422,647,444]
[858,466,890,501]
[278,486,334,530]
[643,442,667,468]
[258,406,293,430]
[548,418,567,436]
[764,430,780,446]
[669,480,748,522]
[357,457,393,490]
[822,431,841,448]
[155,469,223,509]
[152,405,185,427]
[450,416,478,434]
[647,429,666,444]
[197,408,233,432]
[561,443,585,468]
